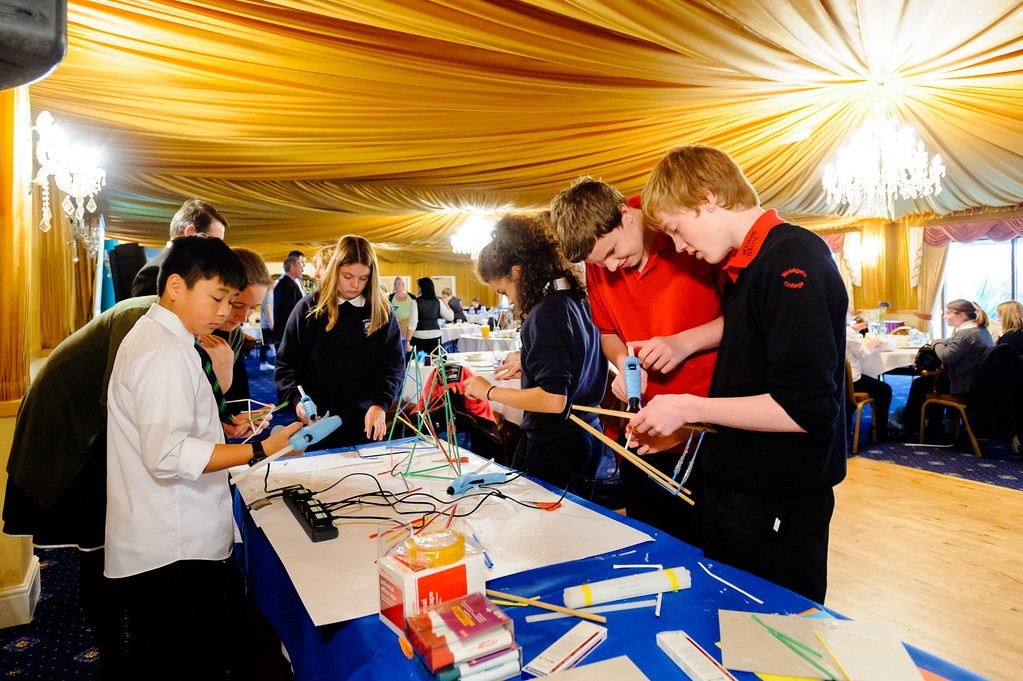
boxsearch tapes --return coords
[406,529,466,567]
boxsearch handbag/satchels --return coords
[913,343,942,372]
[405,388,521,471]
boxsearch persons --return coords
[494,350,522,380]
[467,298,487,314]
[891,299,1023,447]
[846,310,868,332]
[406,277,454,366]
[274,235,405,453]
[3,199,307,681]
[845,323,901,442]
[626,144,849,606]
[463,213,608,502]
[386,276,417,355]
[495,289,509,308]
[552,173,725,543]
[442,288,468,345]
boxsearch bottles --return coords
[480,319,490,338]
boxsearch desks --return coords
[231,435,984,681]
[402,313,525,468]
[861,333,922,383]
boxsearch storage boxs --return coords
[379,546,485,637]
[518,620,607,681]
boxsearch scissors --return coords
[520,500,562,512]
[431,456,469,462]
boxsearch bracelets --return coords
[247,441,268,468]
[486,386,498,401]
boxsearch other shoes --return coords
[923,423,945,441]
[887,427,920,443]
[260,362,275,371]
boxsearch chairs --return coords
[841,327,982,458]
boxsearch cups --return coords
[457,319,462,326]
[481,306,486,314]
[437,319,446,327]
[493,327,500,338]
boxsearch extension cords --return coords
[282,484,339,543]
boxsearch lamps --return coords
[451,212,495,260]
[27,110,111,276]
[820,84,946,222]
